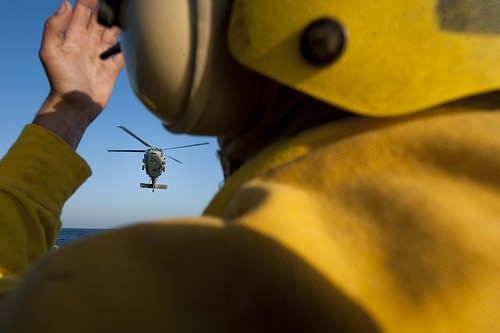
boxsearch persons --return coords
[0,0,500,333]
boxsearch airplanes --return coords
[107,125,210,193]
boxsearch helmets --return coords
[215,2,500,121]
